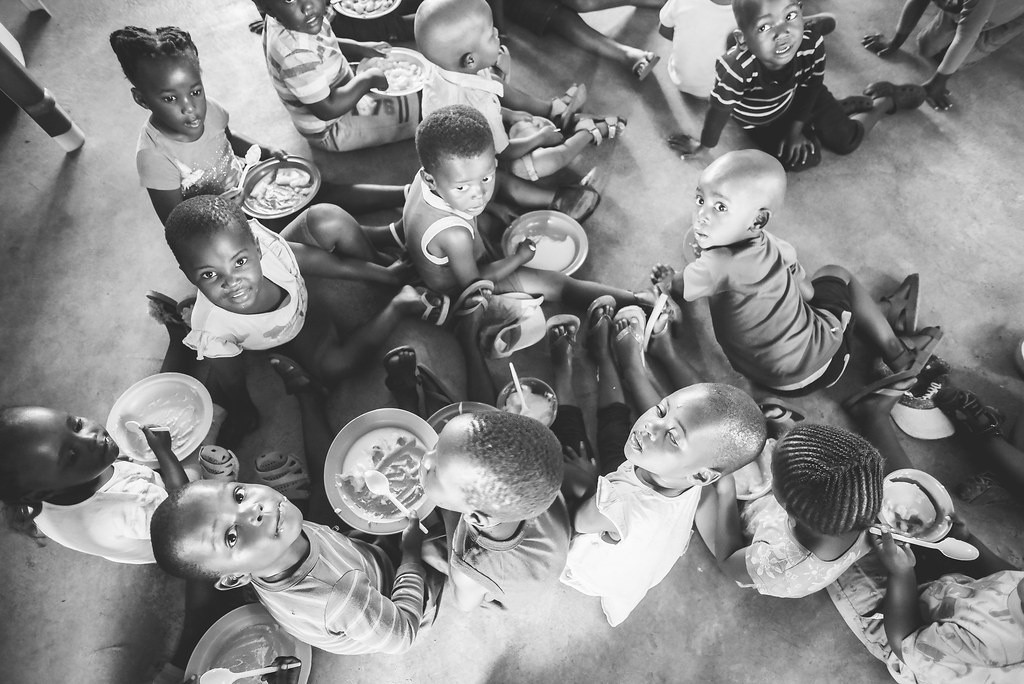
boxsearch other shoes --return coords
[839,95,874,117]
[802,14,837,38]
[863,81,928,116]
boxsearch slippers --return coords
[612,304,646,375]
[544,315,581,362]
[145,288,330,404]
[846,368,918,410]
[642,291,673,353]
[632,50,660,81]
[411,280,494,331]
[384,345,462,407]
[550,183,601,224]
[581,296,616,356]
[760,397,807,425]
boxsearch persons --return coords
[758,370,1024,684]
[862,0,1024,110]
[610,296,883,598]
[413,0,628,181]
[151,345,448,655]
[152,444,311,684]
[683,148,943,398]
[668,0,926,172]
[0,287,260,564]
[402,104,683,340]
[421,279,571,617]
[165,195,450,382]
[546,295,767,628]
[249,0,668,152]
[933,387,1024,506]
[110,25,413,250]
[659,0,835,99]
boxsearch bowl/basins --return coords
[733,445,774,501]
[497,377,558,428]
[509,116,557,139]
[877,468,954,543]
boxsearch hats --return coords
[887,384,958,441]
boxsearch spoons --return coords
[199,662,301,684]
[237,144,261,189]
[510,234,537,253]
[509,362,532,415]
[365,469,428,534]
[869,527,979,561]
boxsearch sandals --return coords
[549,81,588,128]
[196,444,311,507]
[883,324,945,379]
[565,112,628,143]
[938,386,1007,441]
[881,272,920,335]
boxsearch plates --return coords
[106,372,214,469]
[330,0,402,19]
[184,603,312,684]
[356,47,431,96]
[682,223,701,263]
[125,421,170,432]
[324,408,440,535]
[235,155,321,219]
[426,399,502,434]
[501,209,590,274]
